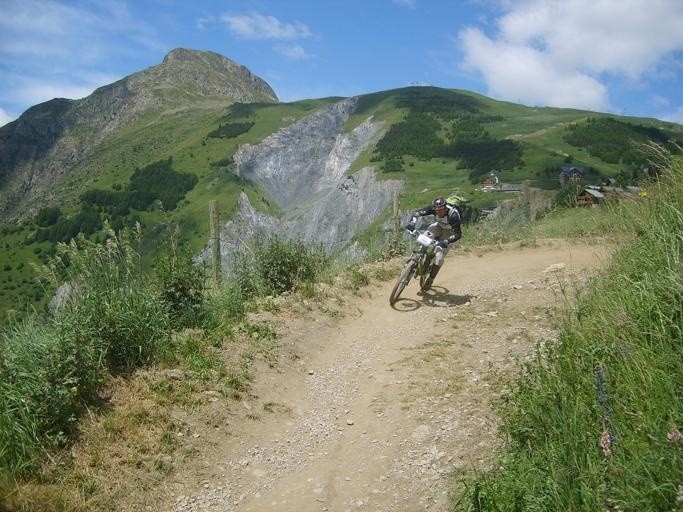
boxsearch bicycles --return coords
[388,225,448,305]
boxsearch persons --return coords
[406,196,463,291]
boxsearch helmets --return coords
[432,197,447,211]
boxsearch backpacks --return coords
[445,195,467,224]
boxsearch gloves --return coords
[405,222,416,232]
[439,240,449,249]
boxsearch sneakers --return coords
[422,278,433,291]
